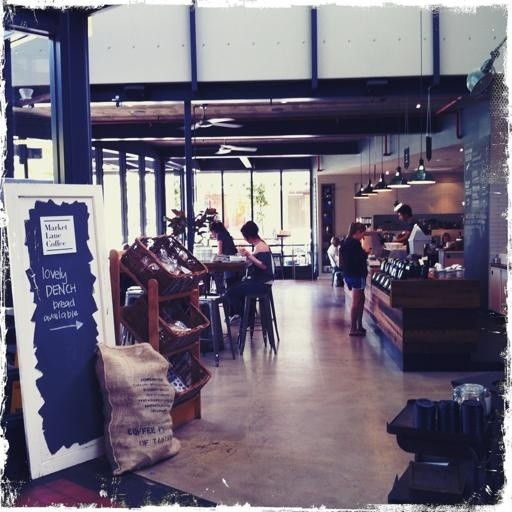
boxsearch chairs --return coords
[272,252,286,280]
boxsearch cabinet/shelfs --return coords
[362,232,483,372]
[110,236,211,429]
[488,262,510,317]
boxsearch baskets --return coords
[119,234,210,296]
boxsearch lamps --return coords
[361,104,376,195]
[373,112,391,192]
[387,104,411,188]
[466,60,496,97]
[406,100,436,184]
[353,127,368,199]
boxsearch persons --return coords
[327,236,341,268]
[438,233,452,249]
[339,222,372,337]
[210,222,246,287]
[393,204,428,255]
[224,221,275,324]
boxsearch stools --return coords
[196,295,236,368]
[239,284,280,355]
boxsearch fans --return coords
[182,105,241,131]
[207,141,257,154]
